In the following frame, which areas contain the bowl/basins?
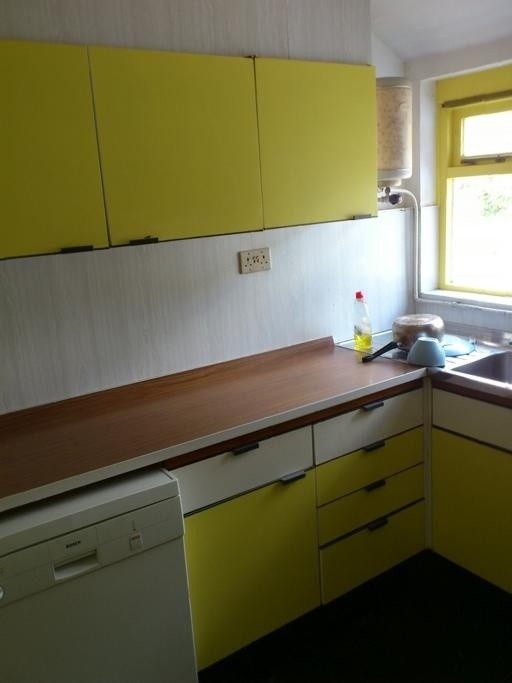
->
[407,337,446,367]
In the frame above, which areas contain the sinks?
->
[450,349,512,385]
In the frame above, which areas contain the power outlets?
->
[239,247,271,274]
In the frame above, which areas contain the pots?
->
[362,313,452,362]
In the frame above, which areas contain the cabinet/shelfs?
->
[312,379,426,604]
[0,43,265,259]
[423,377,510,595]
[253,55,379,230]
[171,421,322,674]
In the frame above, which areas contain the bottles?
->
[352,291,373,350]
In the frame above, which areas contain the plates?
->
[442,332,475,357]
